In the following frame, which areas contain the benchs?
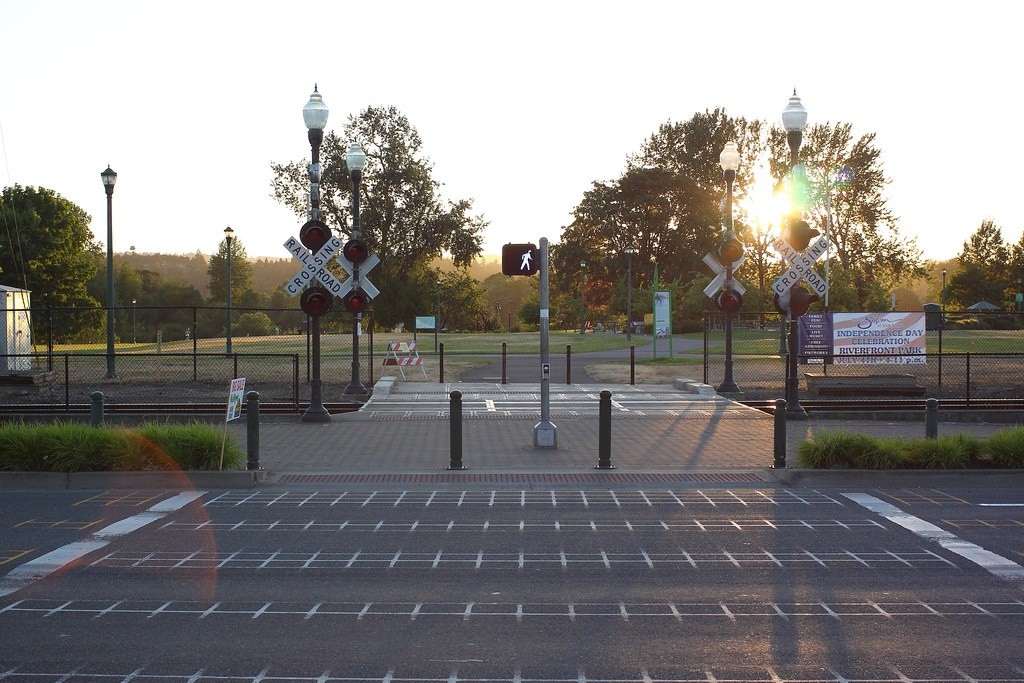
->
[803,372,927,399]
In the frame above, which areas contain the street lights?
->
[579,260,587,334]
[99,163,119,378]
[436,279,442,331]
[223,225,235,359]
[941,268,947,325]
[782,85,808,419]
[302,82,332,423]
[344,135,367,395]
[718,136,742,392]
[132,297,137,344]
[625,243,636,385]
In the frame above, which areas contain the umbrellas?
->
[966,299,1001,310]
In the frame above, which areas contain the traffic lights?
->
[715,289,742,312]
[500,242,539,277]
[718,239,743,263]
[790,221,820,251]
[791,286,820,317]
[343,239,368,262]
[301,287,333,318]
[299,220,334,251]
[343,290,367,314]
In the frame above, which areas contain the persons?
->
[184,328,190,340]
